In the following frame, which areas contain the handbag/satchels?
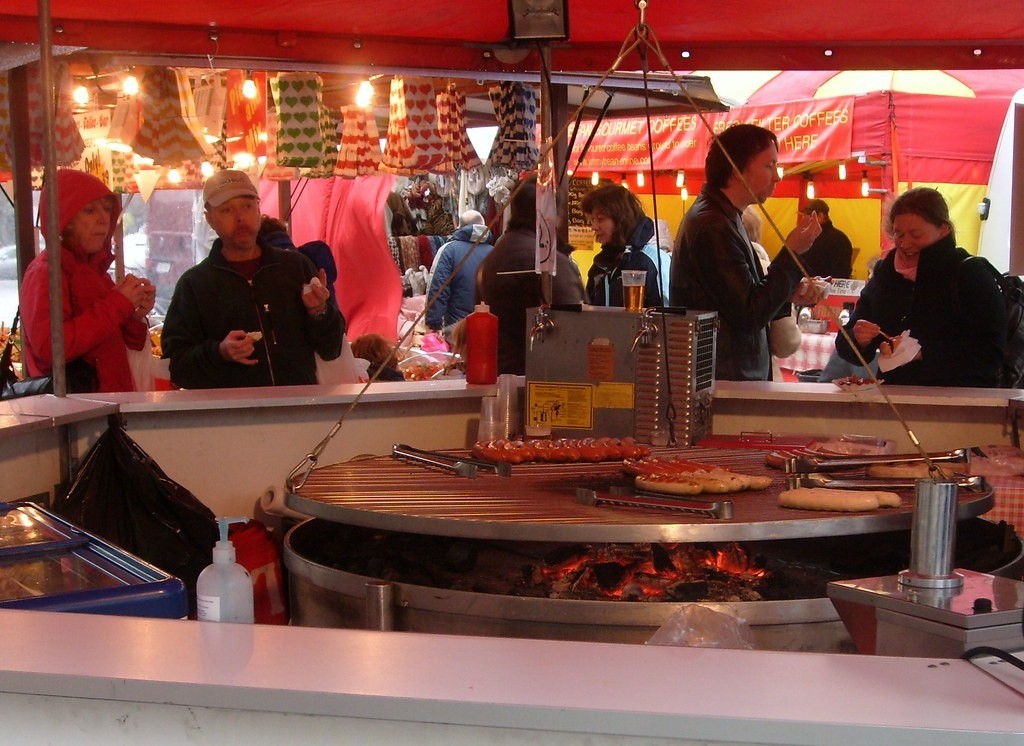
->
[0,342,97,401]
[954,256,1024,389]
[126,329,154,393]
[314,333,371,384]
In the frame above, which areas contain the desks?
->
[774,330,842,376]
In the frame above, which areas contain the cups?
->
[477,374,519,442]
[621,270,648,314]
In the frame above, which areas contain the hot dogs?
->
[801,275,832,286]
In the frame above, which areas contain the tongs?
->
[784,446,970,474]
[392,443,512,479]
[574,486,732,520]
[784,474,986,494]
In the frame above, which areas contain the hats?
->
[203,169,258,210]
[797,199,829,215]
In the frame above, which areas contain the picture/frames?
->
[508,0,571,42]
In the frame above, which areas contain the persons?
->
[582,185,671,306]
[472,177,587,375]
[669,125,834,381]
[350,334,405,382]
[741,205,771,275]
[835,187,993,387]
[797,198,853,278]
[160,170,346,388]
[425,210,494,333]
[19,170,156,393]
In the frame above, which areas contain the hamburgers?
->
[880,337,903,356]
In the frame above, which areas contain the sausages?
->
[473,437,953,509]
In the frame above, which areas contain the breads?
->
[245,331,263,343]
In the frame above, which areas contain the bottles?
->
[465,301,499,385]
